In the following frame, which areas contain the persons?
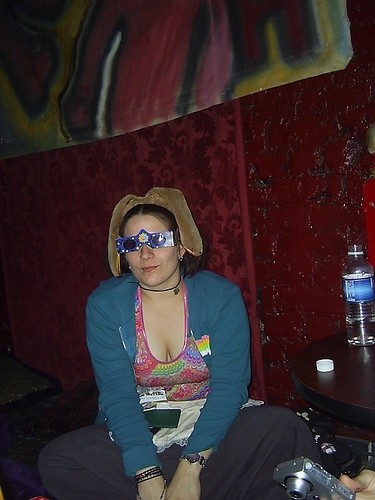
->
[332,458,375,500]
[38,187,321,500]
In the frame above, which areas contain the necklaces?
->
[137,273,182,295]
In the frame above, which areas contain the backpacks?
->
[291,405,363,477]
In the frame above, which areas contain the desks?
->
[290,332,375,445]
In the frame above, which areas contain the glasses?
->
[114,227,178,254]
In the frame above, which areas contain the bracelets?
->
[134,466,167,500]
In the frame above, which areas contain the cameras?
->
[273,456,356,500]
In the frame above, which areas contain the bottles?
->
[342,244,375,346]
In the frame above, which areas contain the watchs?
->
[179,451,207,468]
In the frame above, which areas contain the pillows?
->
[0,354,64,415]
